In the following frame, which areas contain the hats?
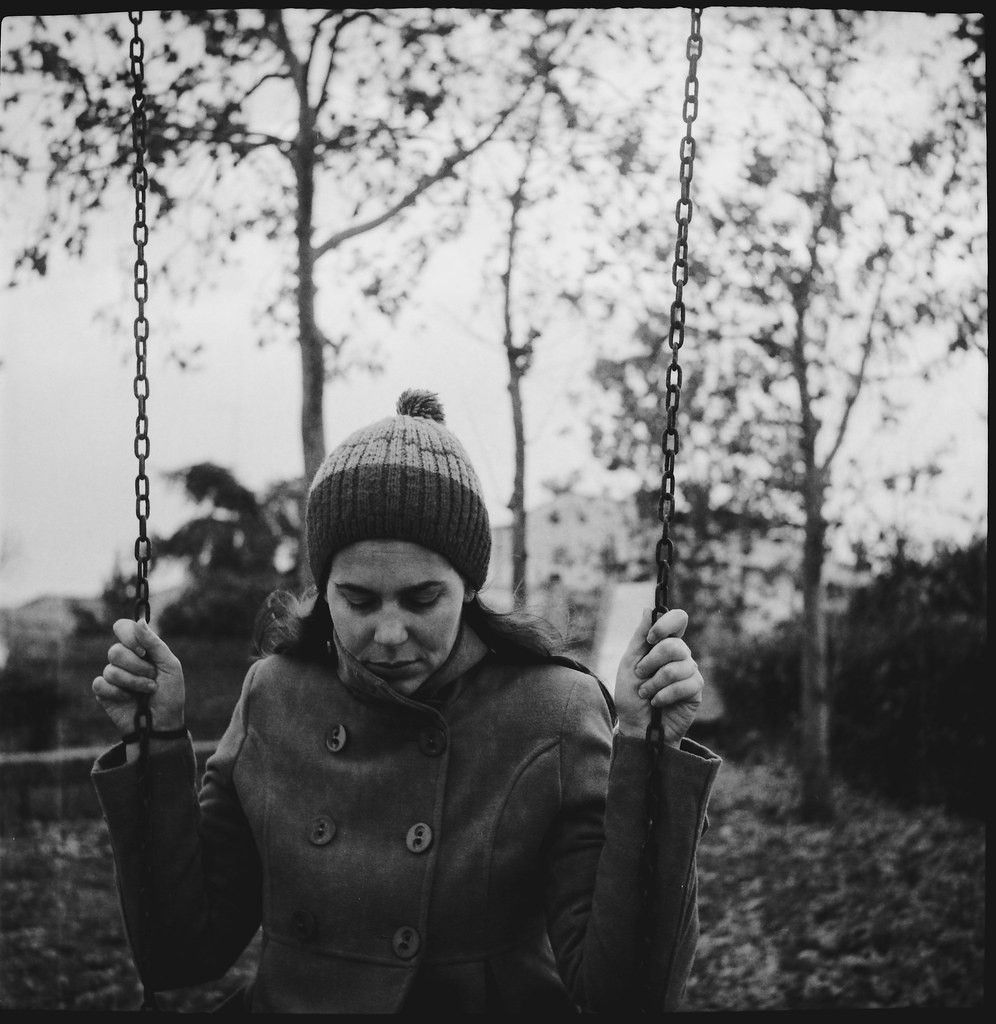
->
[306,389,491,593]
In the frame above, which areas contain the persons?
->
[90,387,722,1024]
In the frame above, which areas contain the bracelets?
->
[120,726,191,744]
